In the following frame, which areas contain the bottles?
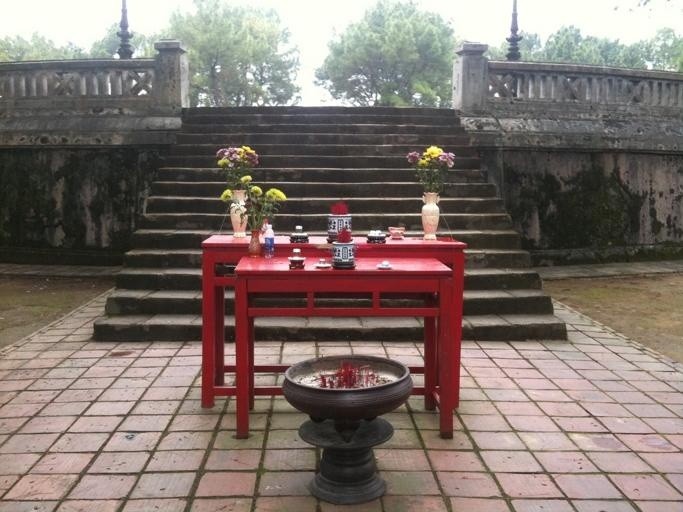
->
[264,225,275,259]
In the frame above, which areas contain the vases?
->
[326,215,354,241]
[247,229,263,258]
[421,192,440,240]
[332,241,357,268]
[229,190,249,238]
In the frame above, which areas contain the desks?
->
[199,230,467,409]
[235,253,458,440]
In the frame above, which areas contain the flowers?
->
[220,175,288,233]
[217,146,260,190]
[330,199,349,214]
[338,226,352,242]
[406,143,457,192]
